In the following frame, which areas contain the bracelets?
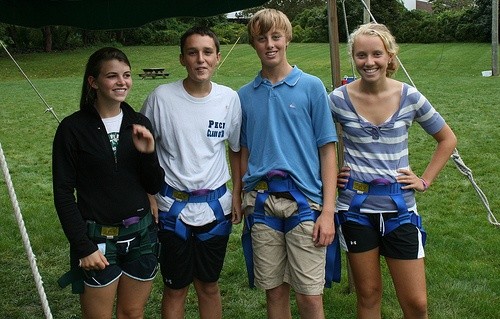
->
[419,176,429,189]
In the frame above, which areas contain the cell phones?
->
[97,243,106,256]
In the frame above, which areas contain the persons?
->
[54,47,166,319]
[328,24,457,319]
[238,9,338,318]
[140,27,241,319]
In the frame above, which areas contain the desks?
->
[142,68,164,73]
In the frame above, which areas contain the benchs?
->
[137,73,169,80]
